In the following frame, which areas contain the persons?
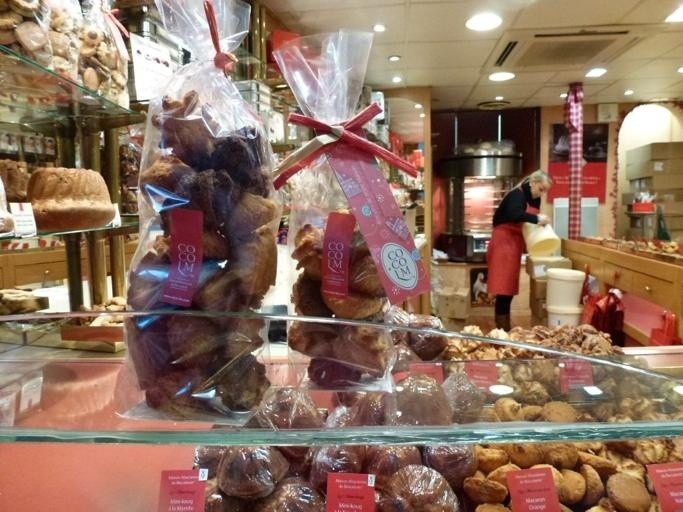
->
[487,169,553,332]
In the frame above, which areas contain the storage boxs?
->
[622,142,683,242]
[526,256,573,327]
[437,286,471,333]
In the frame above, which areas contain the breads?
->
[0,158,30,203]
[27,166,116,233]
[124,89,485,512]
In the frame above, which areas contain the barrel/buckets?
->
[546,267,586,306]
[545,306,583,328]
[522,221,561,257]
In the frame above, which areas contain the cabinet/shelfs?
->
[1,43,148,312]
[561,238,683,346]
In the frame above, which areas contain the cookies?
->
[445,324,683,512]
[0,0,127,92]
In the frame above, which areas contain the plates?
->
[552,148,606,160]
